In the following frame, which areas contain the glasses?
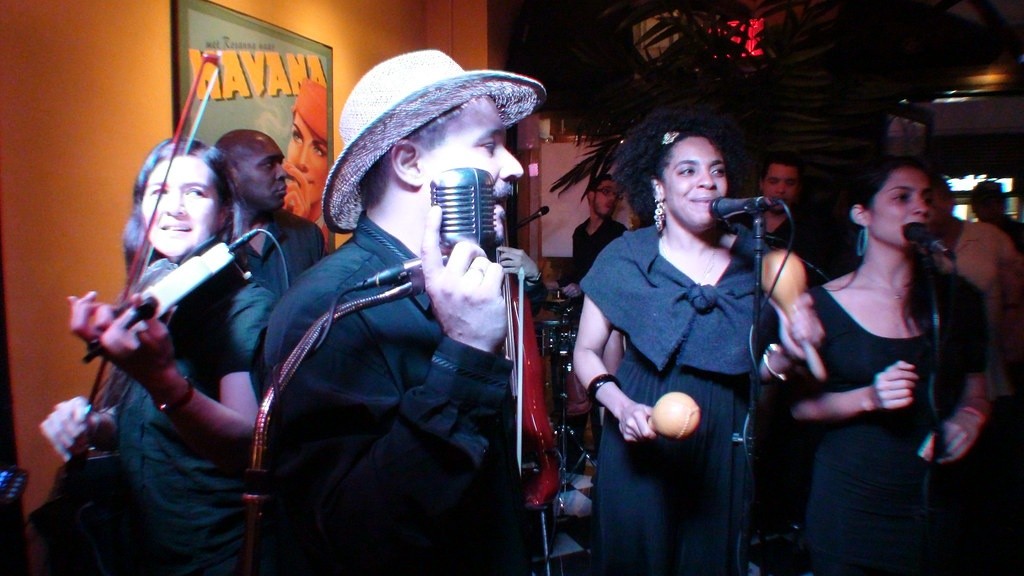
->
[592,187,624,201]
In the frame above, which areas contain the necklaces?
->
[661,233,716,283]
[856,269,910,300]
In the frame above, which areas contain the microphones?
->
[708,195,781,220]
[515,205,550,231]
[355,168,496,292]
[903,221,958,262]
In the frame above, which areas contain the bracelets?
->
[956,404,987,420]
[585,374,621,408]
[150,375,193,414]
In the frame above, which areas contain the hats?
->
[321,49,545,234]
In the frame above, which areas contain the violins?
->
[80,241,235,365]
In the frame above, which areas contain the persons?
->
[39,135,278,576]
[213,130,327,301]
[498,115,1024,576]
[249,50,547,576]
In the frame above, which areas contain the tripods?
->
[543,357,597,525]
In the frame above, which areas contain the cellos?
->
[500,263,564,576]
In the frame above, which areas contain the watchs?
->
[528,271,543,284]
